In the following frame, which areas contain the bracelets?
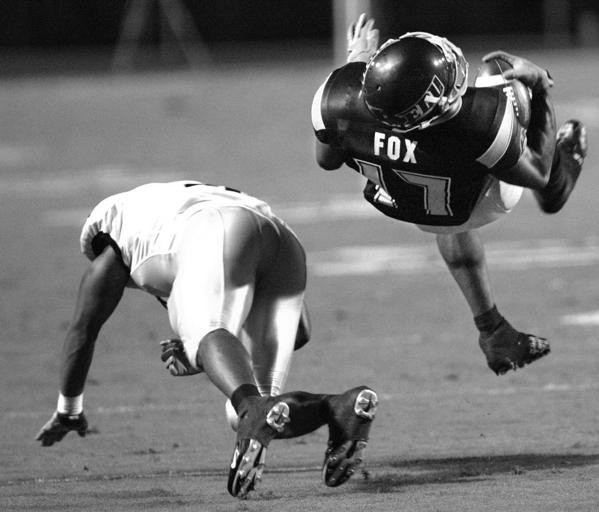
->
[56,388,84,416]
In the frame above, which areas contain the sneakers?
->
[320,384,381,487]
[478,317,551,377]
[225,393,291,500]
[530,119,589,215]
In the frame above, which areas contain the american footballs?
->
[474,58,532,129]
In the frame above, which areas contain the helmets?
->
[360,36,456,132]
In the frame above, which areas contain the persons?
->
[308,10,590,377]
[34,179,379,500]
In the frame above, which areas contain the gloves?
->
[34,393,90,449]
[481,48,553,93]
[159,340,204,378]
[345,13,381,66]
